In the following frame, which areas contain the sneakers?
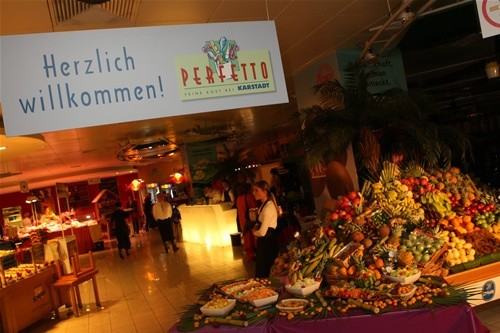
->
[165,244,179,253]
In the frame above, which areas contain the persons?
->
[109,195,138,259]
[144,168,302,278]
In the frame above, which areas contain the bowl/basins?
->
[285,281,320,298]
[247,291,279,309]
[385,269,421,283]
[200,298,236,317]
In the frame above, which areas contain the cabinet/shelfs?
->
[0,263,58,333]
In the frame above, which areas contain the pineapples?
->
[429,162,489,207]
[373,243,415,270]
[352,255,376,287]
[388,223,406,248]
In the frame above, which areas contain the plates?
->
[275,298,309,313]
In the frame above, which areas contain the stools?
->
[50,250,101,318]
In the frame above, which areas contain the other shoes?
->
[120,252,129,259]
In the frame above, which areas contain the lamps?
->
[486,58,500,79]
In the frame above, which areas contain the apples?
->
[326,191,360,222]
[399,176,440,200]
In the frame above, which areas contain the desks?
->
[167,277,491,332]
[48,224,98,254]
